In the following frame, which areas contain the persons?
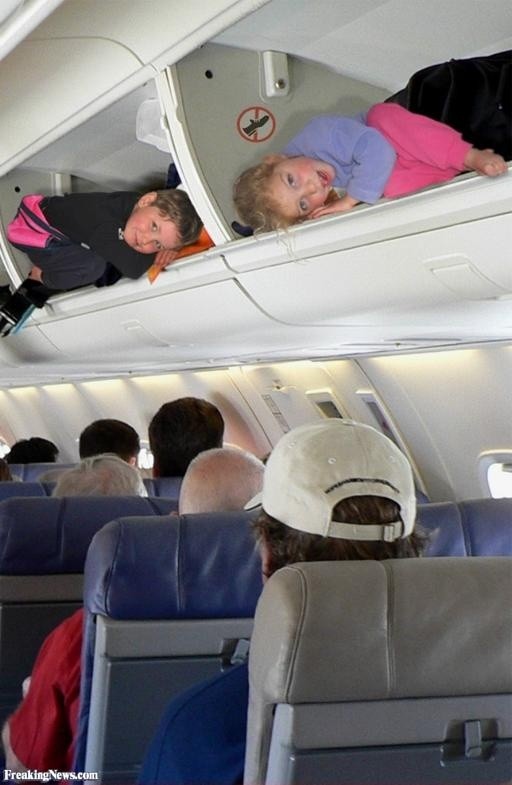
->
[9,438,58,469]
[1,189,200,335]
[148,397,225,477]
[3,445,266,784]
[80,418,141,472]
[234,48,512,227]
[51,455,146,501]
[144,418,421,785]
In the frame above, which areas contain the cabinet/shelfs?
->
[167,2,512,246]
[0,69,219,300]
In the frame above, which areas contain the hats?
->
[242,418,418,542]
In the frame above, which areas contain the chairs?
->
[0,495,188,721]
[74,495,511,785]
[241,558,512,785]
